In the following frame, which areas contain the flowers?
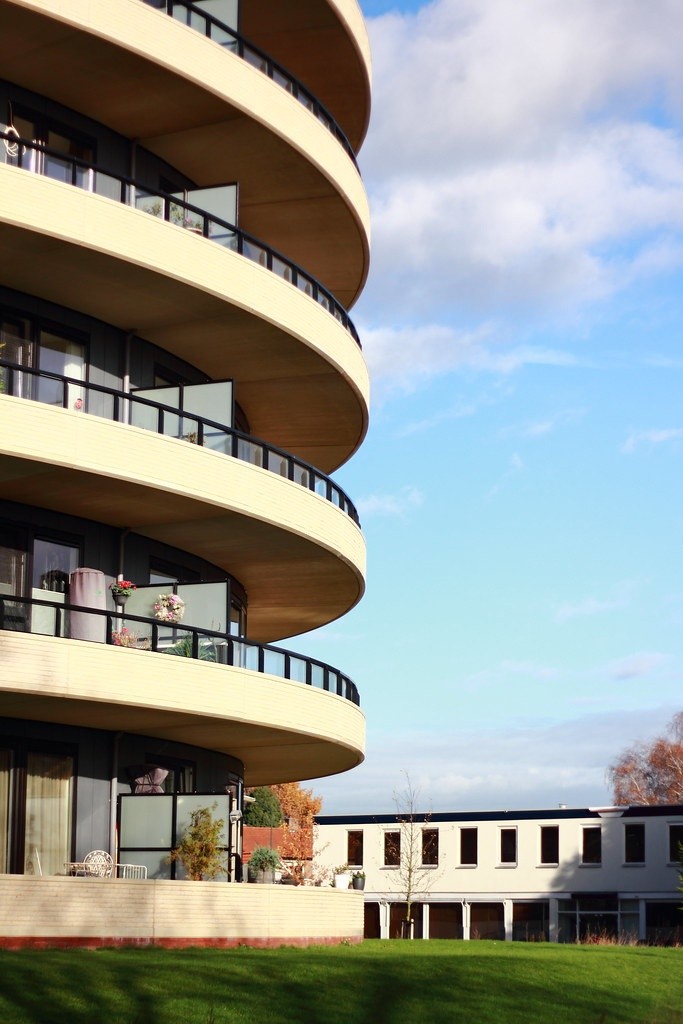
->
[152,593,185,624]
[110,580,136,597]
[110,625,152,650]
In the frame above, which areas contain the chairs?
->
[83,850,112,878]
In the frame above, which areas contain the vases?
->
[112,594,129,605]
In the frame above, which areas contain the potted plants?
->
[334,866,350,889]
[185,220,202,236]
[353,870,365,891]
[281,871,300,886]
